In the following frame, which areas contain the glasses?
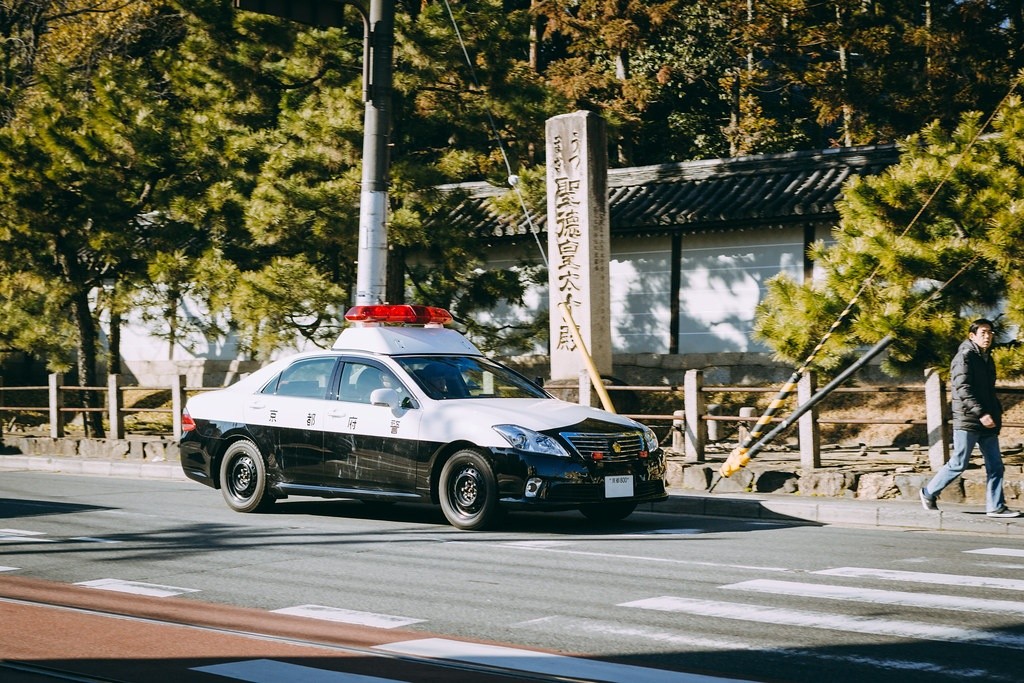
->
[973,331,995,336]
[431,376,447,382]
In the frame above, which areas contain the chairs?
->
[354,367,385,404]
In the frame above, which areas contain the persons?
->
[919,318,1021,518]
[366,364,458,409]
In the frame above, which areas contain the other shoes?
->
[919,488,939,510]
[987,508,1021,518]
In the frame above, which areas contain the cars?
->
[179,303,669,532]
